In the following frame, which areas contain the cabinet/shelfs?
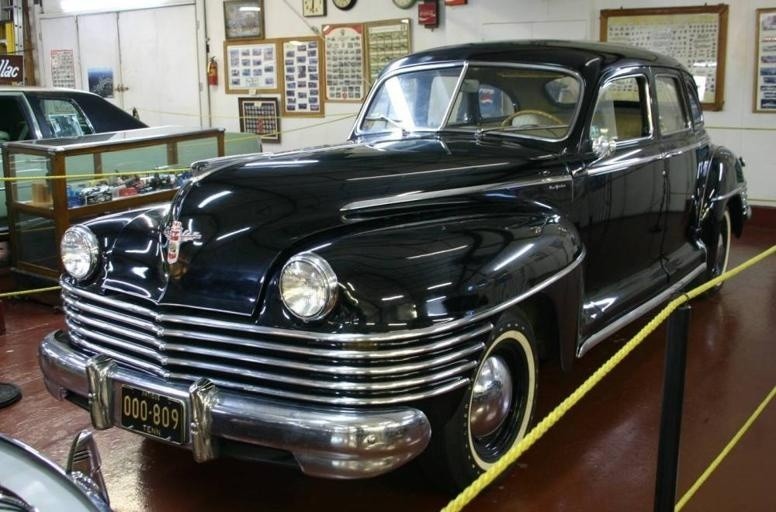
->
[3,124,225,312]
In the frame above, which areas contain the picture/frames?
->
[599,3,729,112]
[750,8,776,114]
[223,0,413,144]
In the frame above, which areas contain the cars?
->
[35,32,758,486]
[0,86,266,243]
[0,429,116,512]
